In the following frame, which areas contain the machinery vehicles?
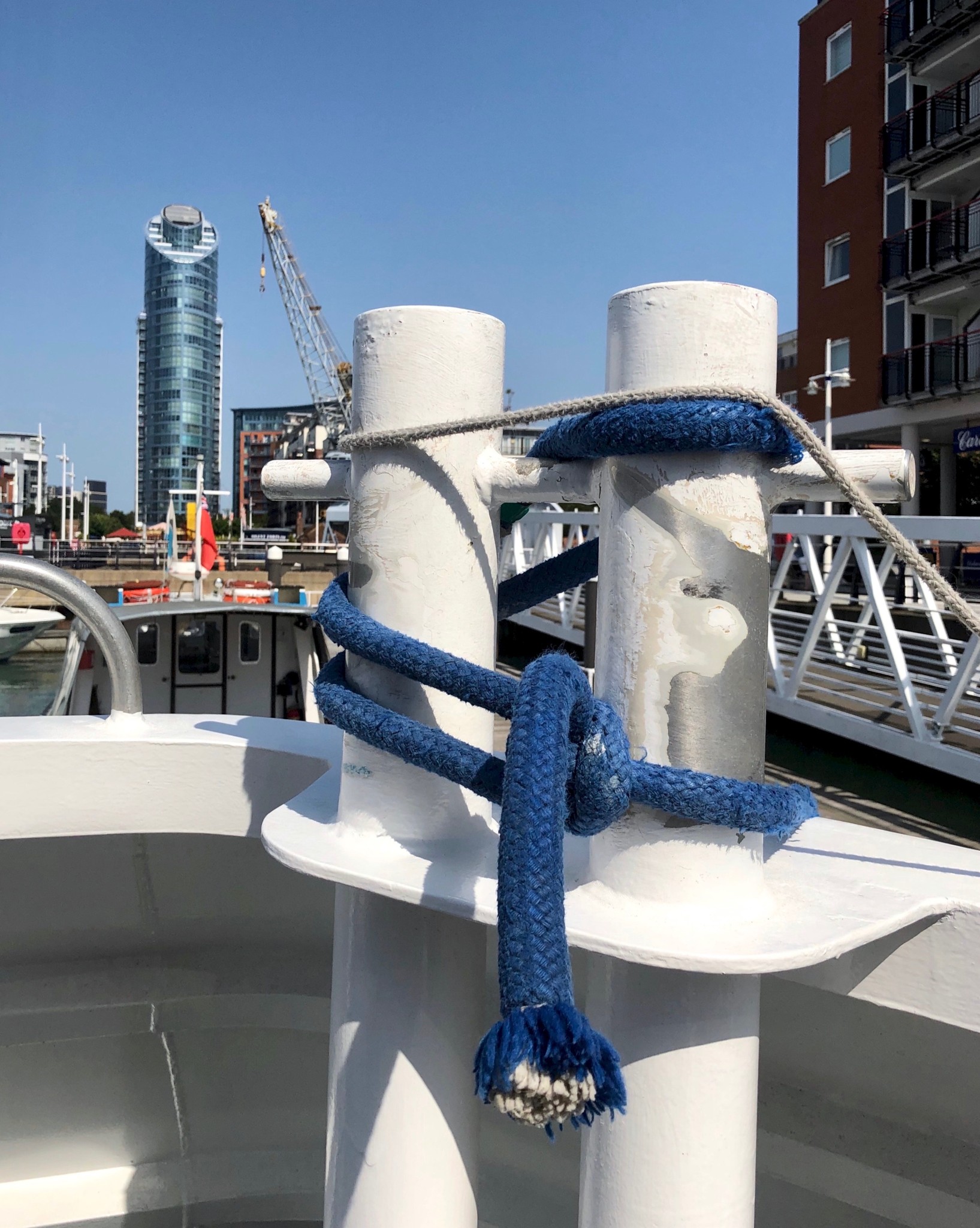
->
[258,195,352,447]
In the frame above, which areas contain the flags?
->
[196,482,218,572]
[164,498,178,574]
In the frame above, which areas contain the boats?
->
[168,559,210,583]
[0,606,66,662]
